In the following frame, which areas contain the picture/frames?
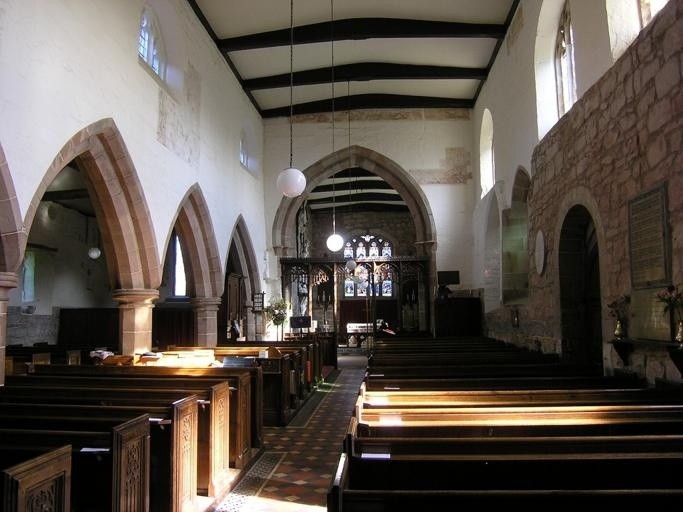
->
[627,178,670,289]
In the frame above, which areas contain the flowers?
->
[601,287,683,319]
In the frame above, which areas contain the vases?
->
[612,320,683,343]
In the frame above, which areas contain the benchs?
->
[325,327,682,512]
[1,329,341,512]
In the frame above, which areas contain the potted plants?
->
[267,301,287,325]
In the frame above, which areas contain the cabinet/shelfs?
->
[57,273,246,351]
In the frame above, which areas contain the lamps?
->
[88,212,102,259]
[275,0,357,276]
